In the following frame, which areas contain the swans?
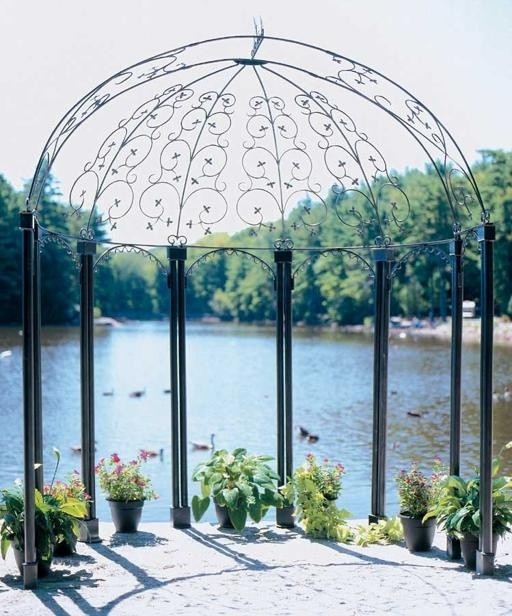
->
[140,447,164,458]
[190,431,217,450]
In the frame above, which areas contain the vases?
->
[54,538,73,556]
[397,511,435,550]
[106,497,144,534]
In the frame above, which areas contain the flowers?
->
[394,453,448,520]
[94,449,160,502]
[42,447,94,509]
[304,451,346,500]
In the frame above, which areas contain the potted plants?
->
[0,482,92,579]
[193,448,296,530]
[421,438,512,572]
